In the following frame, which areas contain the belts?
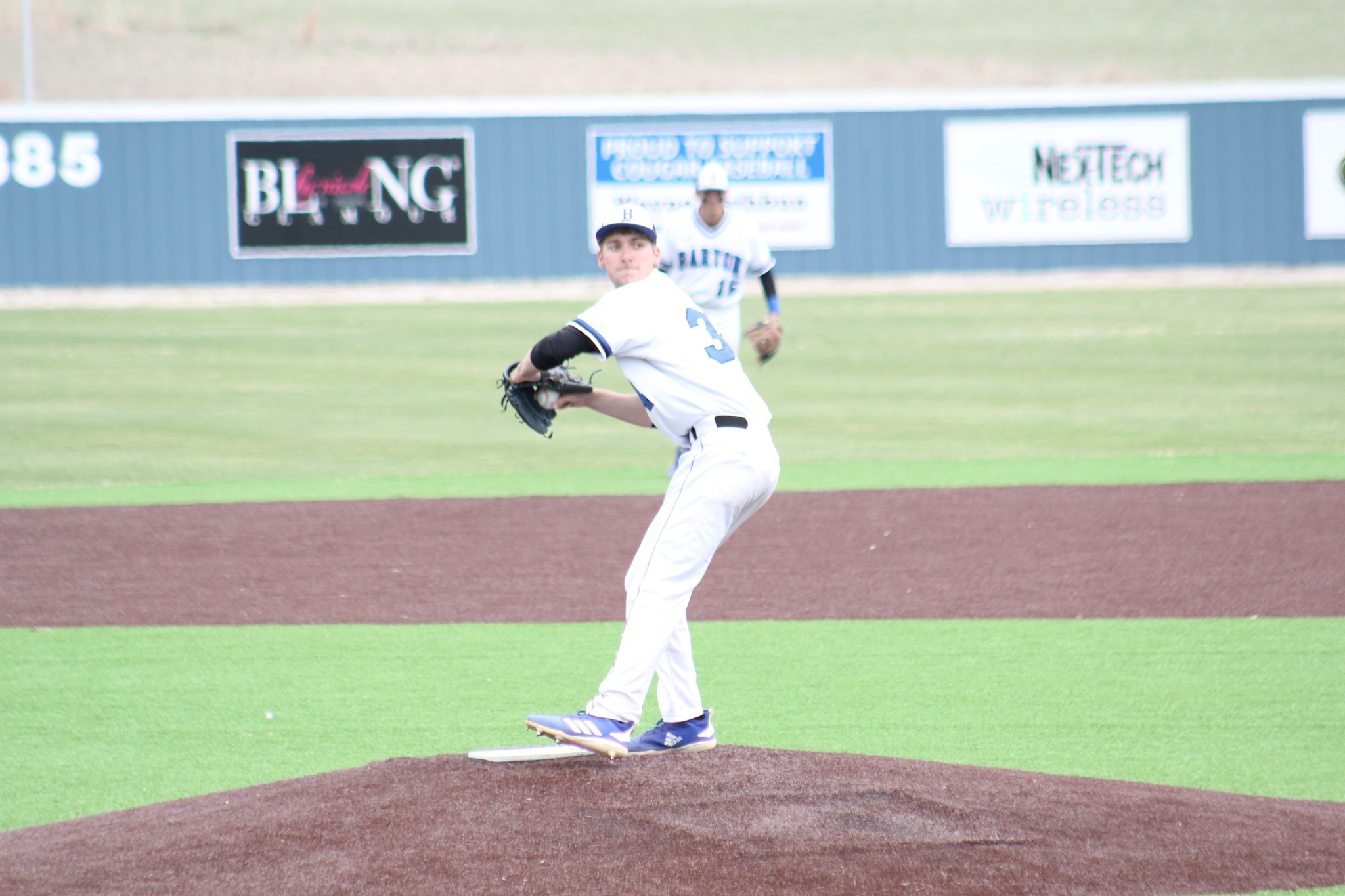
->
[686,415,747,443]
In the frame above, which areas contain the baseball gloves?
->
[744,320,783,364]
[502,361,594,435]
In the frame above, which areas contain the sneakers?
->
[628,709,716,755]
[526,710,638,759]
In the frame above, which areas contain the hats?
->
[596,203,657,247]
[695,161,729,192]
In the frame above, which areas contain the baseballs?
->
[537,386,561,410]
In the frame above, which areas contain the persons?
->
[655,160,780,376]
[500,203,779,762]
